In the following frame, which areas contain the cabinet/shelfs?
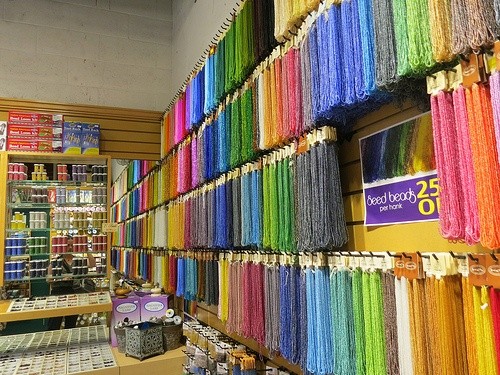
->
[1,149,112,288]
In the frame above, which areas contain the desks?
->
[0,291,113,327]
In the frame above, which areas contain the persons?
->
[48,251,97,332]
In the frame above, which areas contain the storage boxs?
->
[109,287,184,362]
[0,111,100,154]
